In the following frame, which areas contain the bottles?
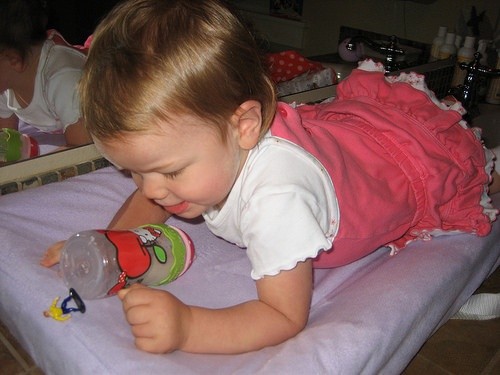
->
[431,26,448,59]
[59,223,195,299]
[454,35,462,49]
[452,37,477,88]
[441,33,456,60]
[0,128,40,164]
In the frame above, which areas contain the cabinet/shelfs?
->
[237,8,341,57]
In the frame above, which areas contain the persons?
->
[0,0,95,154]
[43,288,85,322]
[42,0,500,355]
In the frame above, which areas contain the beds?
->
[18,25,433,156]
[0,58,500,374]
